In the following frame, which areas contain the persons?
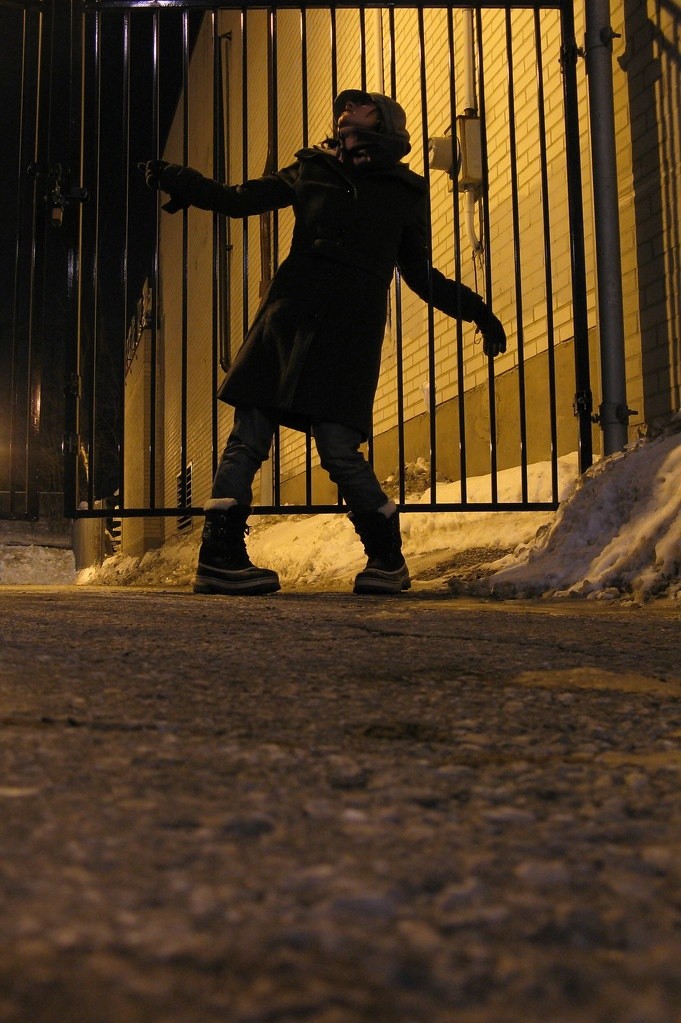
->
[138,90,506,596]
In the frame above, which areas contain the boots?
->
[347,502,412,594]
[194,499,281,594]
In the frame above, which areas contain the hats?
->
[333,89,410,162]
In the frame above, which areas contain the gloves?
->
[474,302,506,356]
[138,158,187,194]
